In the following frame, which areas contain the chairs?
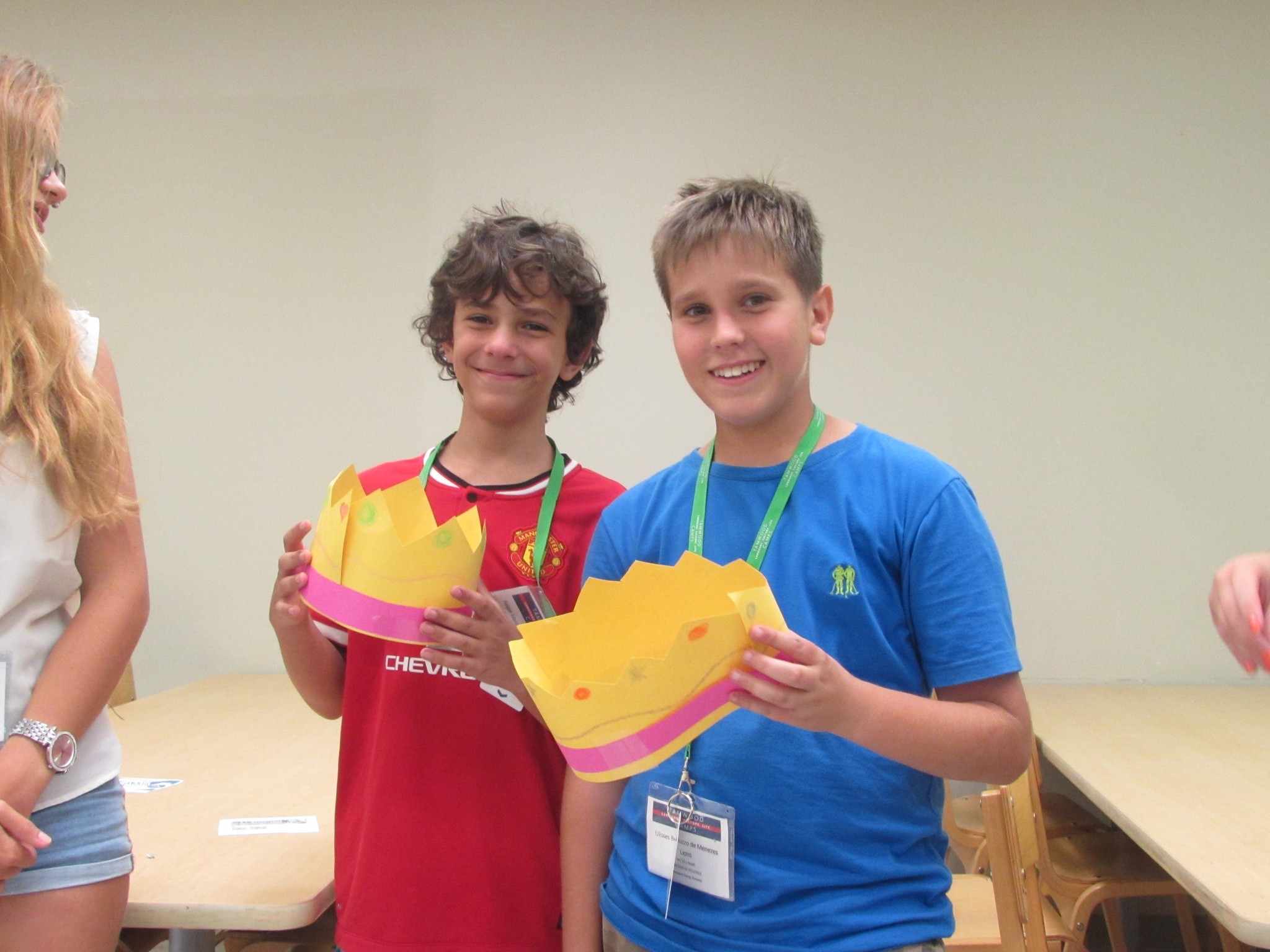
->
[943,730,1202,952]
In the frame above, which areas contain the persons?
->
[561,177,1031,952]
[269,216,629,952]
[0,54,150,952]
[1209,553,1270,675]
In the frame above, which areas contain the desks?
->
[107,671,340,952]
[1020,683,1270,951]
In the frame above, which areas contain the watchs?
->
[8,718,76,775]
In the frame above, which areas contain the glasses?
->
[28,128,65,208]
